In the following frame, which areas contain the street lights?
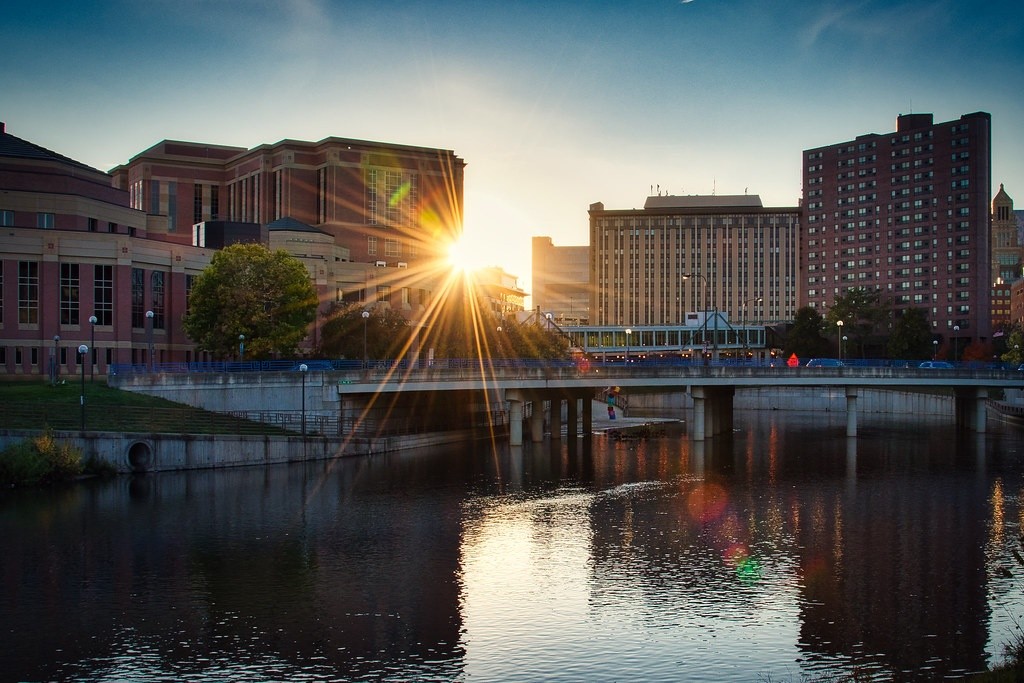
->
[78,344,89,431]
[545,312,552,360]
[239,334,244,373]
[842,335,848,360]
[836,320,843,361]
[681,274,708,367]
[299,364,308,435]
[626,329,633,359]
[362,311,369,371]
[145,311,154,366]
[88,316,98,385]
[742,297,763,367]
[954,325,960,362]
[933,340,937,359]
[496,326,503,357]
[54,335,61,384]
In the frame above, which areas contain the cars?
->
[919,361,955,368]
[806,358,846,367]
